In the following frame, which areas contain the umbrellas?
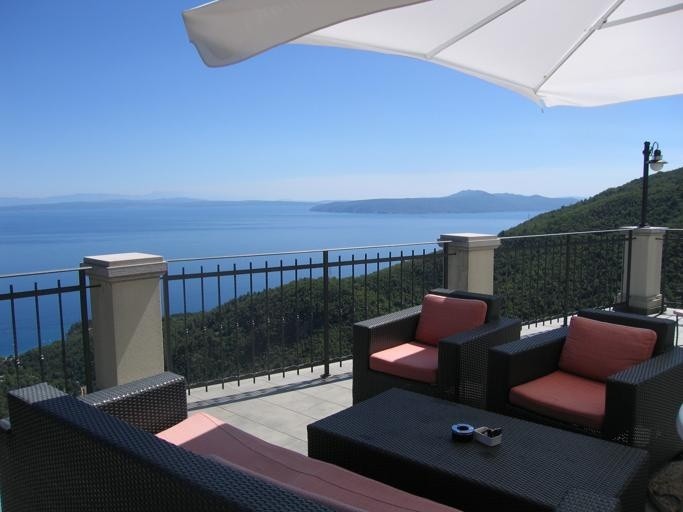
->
[181,0,683,109]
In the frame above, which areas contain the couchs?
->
[490,308,682,474]
[1,372,619,511]
[350,287,520,411]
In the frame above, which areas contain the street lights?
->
[638,141,670,229]
